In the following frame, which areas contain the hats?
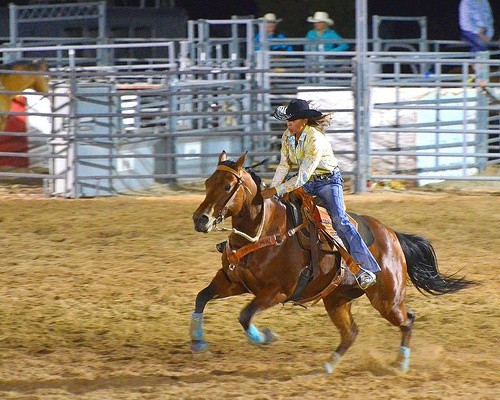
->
[274,99,322,121]
[307,11,334,25]
[258,12,283,23]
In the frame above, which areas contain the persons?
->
[252,13,292,78]
[261,99,381,288]
[459,0,494,60]
[304,11,348,83]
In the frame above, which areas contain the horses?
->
[190,150,484,374]
[0,56,49,130]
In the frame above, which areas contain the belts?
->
[308,168,340,182]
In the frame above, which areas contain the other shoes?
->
[360,272,377,287]
